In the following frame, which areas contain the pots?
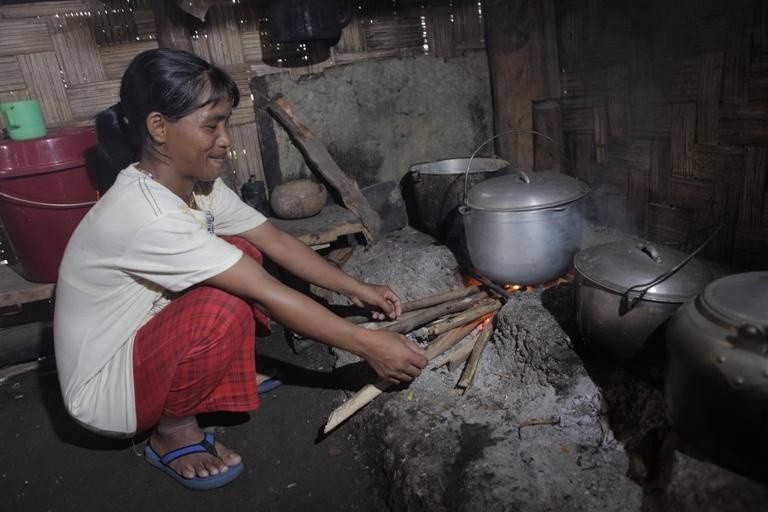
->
[458,127,592,286]
[573,239,714,373]
[662,270,768,486]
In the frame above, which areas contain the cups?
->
[0,98,47,142]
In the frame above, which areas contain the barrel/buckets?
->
[1,125,99,284]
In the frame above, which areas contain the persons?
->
[53,46,431,490]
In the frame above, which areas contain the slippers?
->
[257,367,281,393]
[145,433,245,490]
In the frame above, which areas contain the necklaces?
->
[136,166,197,210]
